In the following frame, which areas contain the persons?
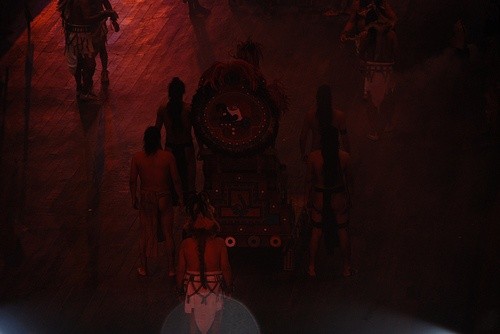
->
[58,0,119,103]
[176,193,237,334]
[130,127,182,277]
[185,0,210,19]
[298,86,351,158]
[350,1,398,142]
[300,128,353,278]
[155,77,206,217]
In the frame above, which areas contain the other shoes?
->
[101,70,110,89]
[168,265,176,277]
[137,267,148,276]
[75,87,82,96]
[79,92,97,100]
